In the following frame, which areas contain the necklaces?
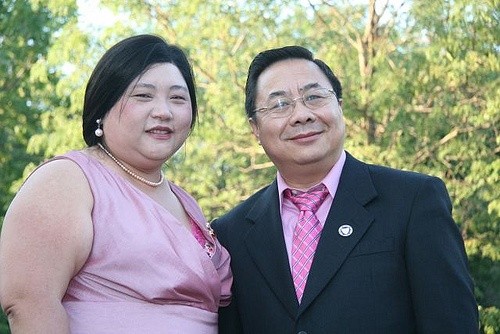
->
[97,141,165,186]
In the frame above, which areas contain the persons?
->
[1,33,234,333]
[207,45,484,334]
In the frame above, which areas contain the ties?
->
[283,182,329,306]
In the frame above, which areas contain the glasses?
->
[252,88,339,117]
